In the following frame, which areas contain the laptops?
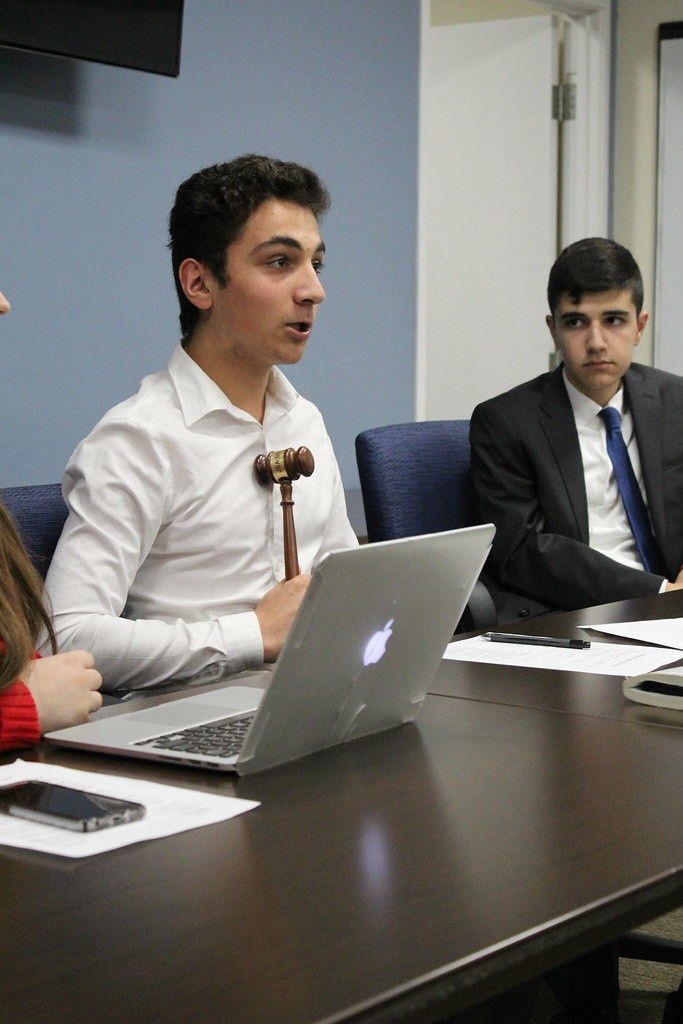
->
[41,522,496,777]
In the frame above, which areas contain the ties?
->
[598,408,664,577]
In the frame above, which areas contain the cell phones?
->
[0,780,146,833]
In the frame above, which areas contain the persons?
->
[0,291,104,747]
[36,153,359,701]
[467,237,683,626]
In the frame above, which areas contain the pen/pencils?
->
[481,632,590,649]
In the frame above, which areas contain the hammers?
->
[253,446,316,581]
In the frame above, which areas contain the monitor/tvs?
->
[0,0,184,77]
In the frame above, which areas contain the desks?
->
[0,588,683,1024]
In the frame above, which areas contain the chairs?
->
[0,484,69,582]
[354,420,501,634]
